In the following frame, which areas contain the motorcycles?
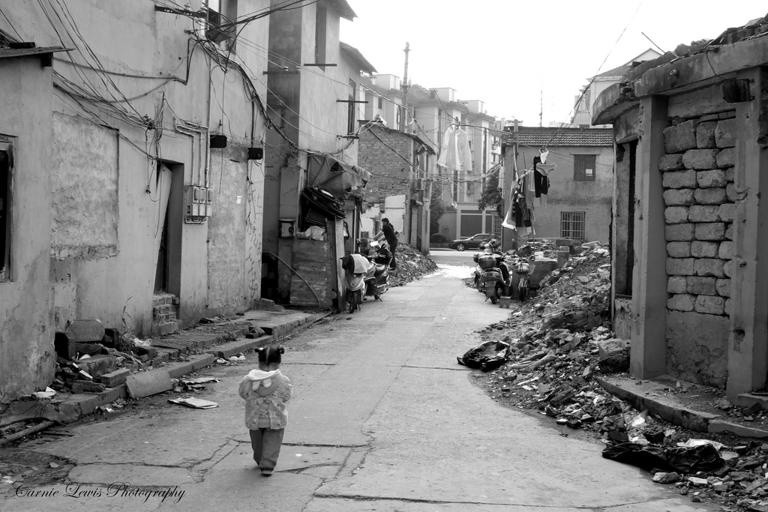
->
[337,236,394,312]
[472,238,535,306]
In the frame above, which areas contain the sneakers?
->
[260,462,275,475]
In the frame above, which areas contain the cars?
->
[449,230,499,252]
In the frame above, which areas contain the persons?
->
[239,346,293,479]
[374,218,399,270]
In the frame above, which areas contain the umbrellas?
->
[342,253,370,273]
